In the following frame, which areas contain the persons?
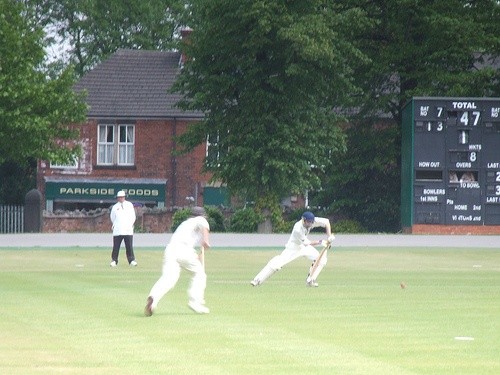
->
[110,191,136,266]
[250,212,332,286]
[144,208,211,316]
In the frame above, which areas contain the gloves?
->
[319,239,327,247]
[328,235,335,242]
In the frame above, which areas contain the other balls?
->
[401,282,407,288]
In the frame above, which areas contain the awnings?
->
[203,187,231,205]
[43,176,167,201]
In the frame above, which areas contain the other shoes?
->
[251,280,257,286]
[130,261,137,266]
[110,261,116,266]
[145,296,154,316]
[306,282,318,287]
[188,301,210,314]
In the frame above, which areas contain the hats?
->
[117,190,125,197]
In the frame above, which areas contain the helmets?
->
[301,212,315,223]
[189,206,204,216]
